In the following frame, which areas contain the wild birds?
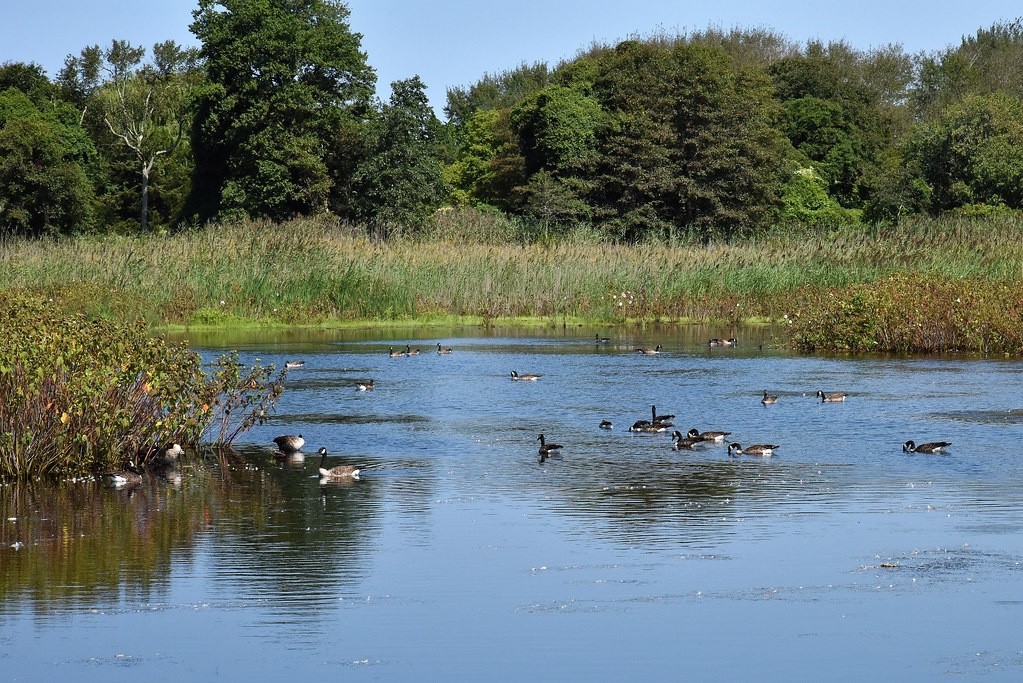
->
[149,443,187,467]
[598,419,614,429]
[816,390,848,402]
[635,344,663,355]
[510,371,543,381]
[285,360,305,368]
[628,404,675,433]
[671,428,732,449]
[903,440,953,455]
[728,442,781,457]
[594,333,610,343]
[708,337,738,347]
[436,342,455,354]
[388,344,422,358]
[102,458,143,484]
[315,447,366,477]
[536,433,565,454]
[761,389,778,404]
[354,379,377,392]
[272,433,306,453]
